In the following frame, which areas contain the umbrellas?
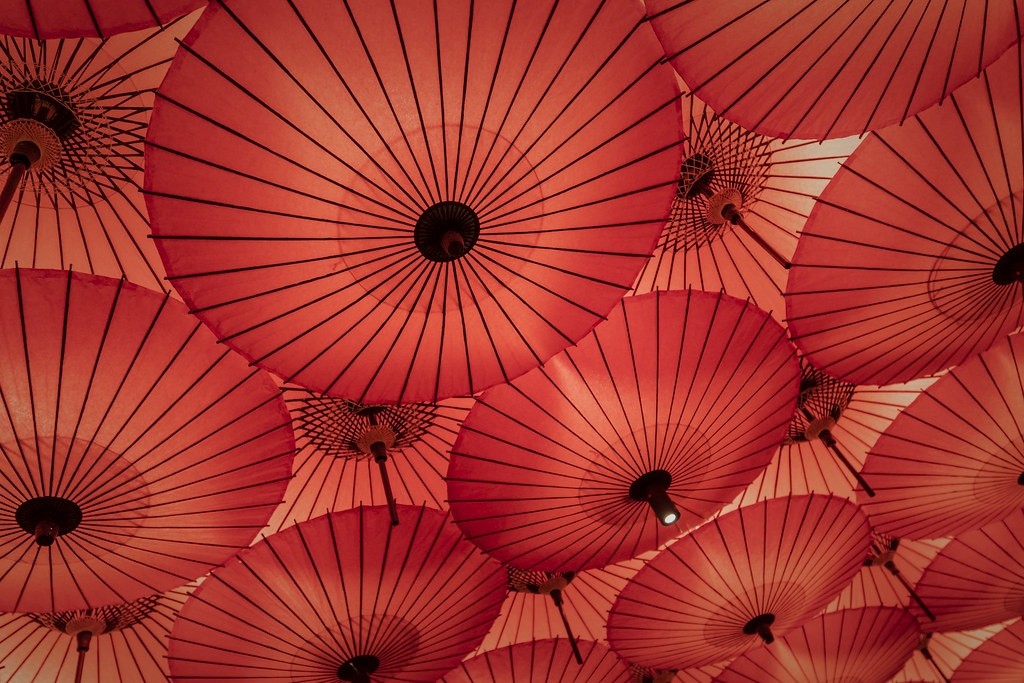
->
[1,370,1024,683]
[144,1,684,404]
[443,285,802,573]
[0,1,210,305]
[623,0,1023,385]
[0,268,295,612]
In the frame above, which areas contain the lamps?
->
[630,467,681,526]
